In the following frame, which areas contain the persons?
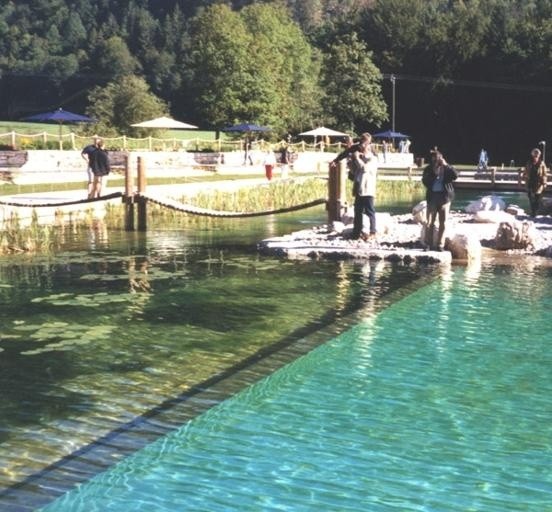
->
[81,137,104,199]
[422,149,458,253]
[345,137,354,161]
[330,132,378,214]
[341,142,378,240]
[396,138,411,153]
[381,140,389,163]
[519,147,550,220]
[242,135,253,165]
[85,140,110,199]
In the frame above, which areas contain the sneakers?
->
[424,244,431,251]
[438,246,444,252]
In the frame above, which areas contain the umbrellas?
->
[17,107,100,140]
[297,125,350,141]
[129,115,199,128]
[222,121,272,136]
[373,128,411,143]
